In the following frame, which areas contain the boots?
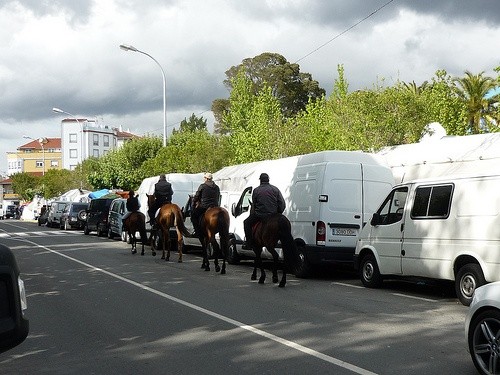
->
[121,218,128,231]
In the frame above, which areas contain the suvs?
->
[60,203,90,230]
[84,199,113,237]
[47,202,66,228]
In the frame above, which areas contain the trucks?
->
[0,203,19,220]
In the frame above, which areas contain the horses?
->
[122,212,147,255]
[146,203,192,263]
[188,194,231,275]
[243,198,301,289]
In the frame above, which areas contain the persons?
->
[241,172,286,250]
[11,207,22,219]
[190,172,220,239]
[122,190,141,232]
[148,173,174,226]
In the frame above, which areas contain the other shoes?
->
[193,232,200,237]
[241,244,253,250]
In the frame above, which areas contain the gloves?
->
[191,202,197,208]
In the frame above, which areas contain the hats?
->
[160,174,166,179]
[259,173,269,182]
[127,190,135,195]
[204,173,212,179]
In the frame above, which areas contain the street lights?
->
[23,135,45,191]
[119,43,167,147]
[51,107,83,190]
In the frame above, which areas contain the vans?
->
[353,174,500,306]
[126,174,218,250]
[178,191,251,259]
[107,198,126,242]
[229,151,399,278]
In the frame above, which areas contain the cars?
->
[464,281,500,375]
[0,244,31,354]
[38,210,50,226]
